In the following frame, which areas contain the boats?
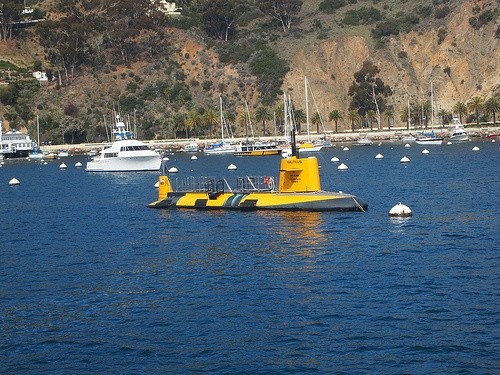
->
[183,143,200,153]
[1,115,42,158]
[84,101,164,171]
[352,137,374,147]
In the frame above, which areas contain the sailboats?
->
[147,94,368,215]
[203,75,336,155]
[390,79,500,146]
